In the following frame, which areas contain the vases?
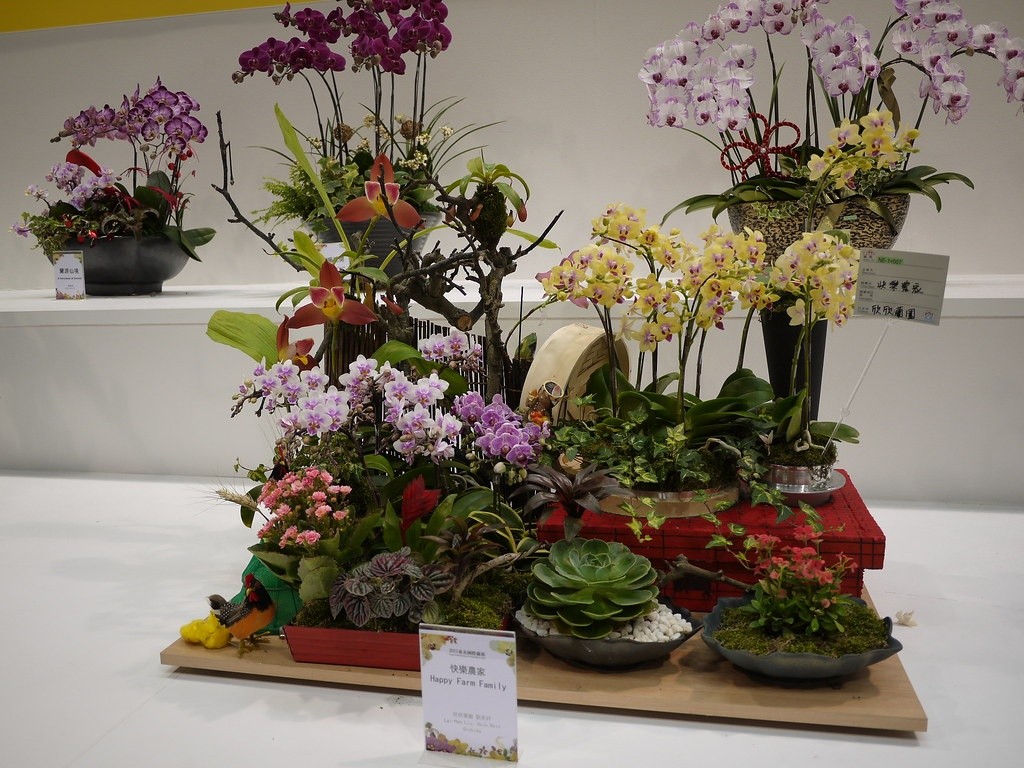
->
[699,592,903,681]
[628,442,730,492]
[305,214,442,292]
[758,463,846,507]
[724,194,911,265]
[45,236,189,297]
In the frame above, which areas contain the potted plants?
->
[511,537,703,672]
[282,461,620,672]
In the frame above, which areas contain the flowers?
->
[206,1,1024,612]
[5,75,217,263]
